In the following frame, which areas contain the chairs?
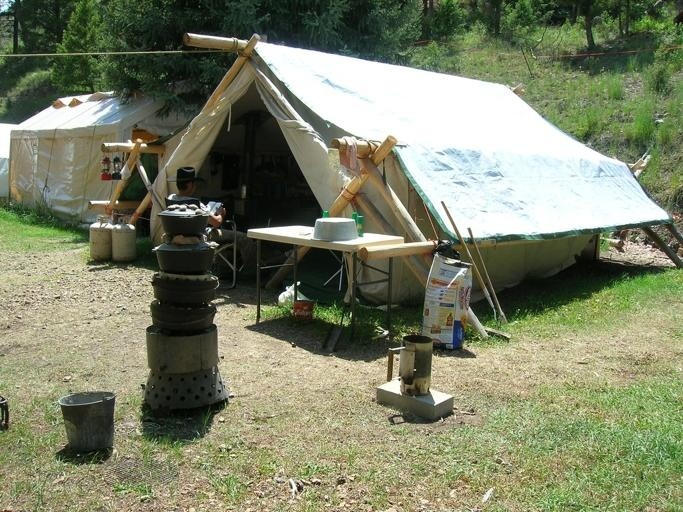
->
[164,198,238,292]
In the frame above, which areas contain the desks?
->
[248,224,405,344]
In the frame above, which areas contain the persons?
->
[165,166,263,278]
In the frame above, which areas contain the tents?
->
[148,39,673,313]
[9,78,217,229]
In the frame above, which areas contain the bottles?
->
[351,212,365,237]
[322,210,329,218]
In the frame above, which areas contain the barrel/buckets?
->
[59,390,116,453]
[294,299,315,322]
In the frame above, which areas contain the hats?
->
[167,167,208,182]
